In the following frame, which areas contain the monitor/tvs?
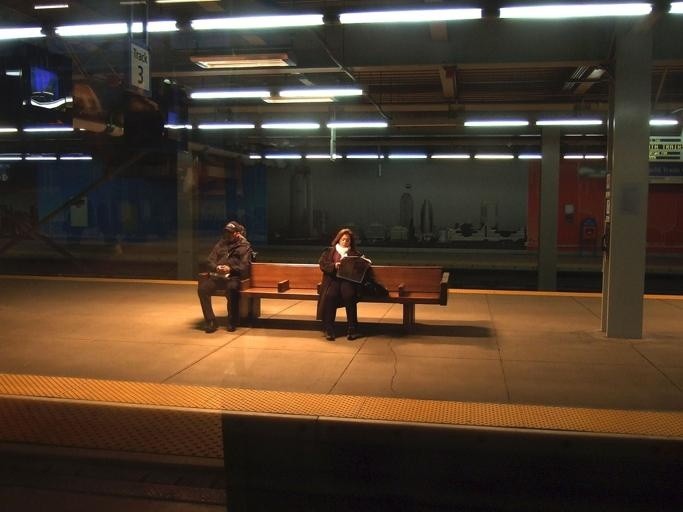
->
[159,83,188,162]
[17,43,72,126]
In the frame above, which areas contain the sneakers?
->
[226,320,235,332]
[324,325,336,341]
[346,325,357,341]
[204,319,219,333]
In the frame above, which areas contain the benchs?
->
[210,262,450,331]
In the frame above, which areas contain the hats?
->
[223,220,241,233]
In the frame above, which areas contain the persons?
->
[197,222,251,332]
[315,228,388,341]
